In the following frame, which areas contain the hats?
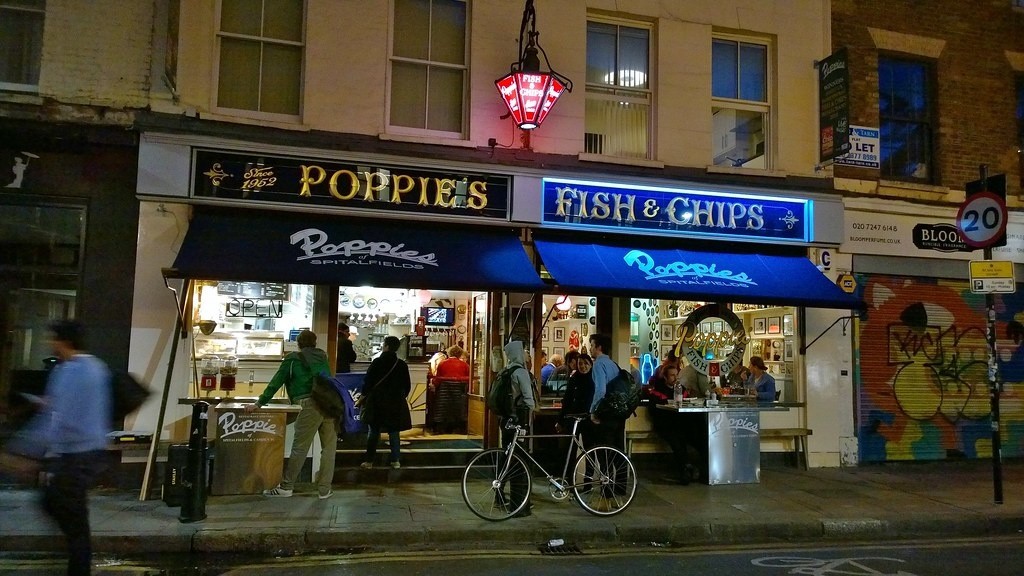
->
[349,326,359,336]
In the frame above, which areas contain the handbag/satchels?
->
[592,360,641,429]
[354,395,366,407]
[298,351,346,418]
[112,370,150,420]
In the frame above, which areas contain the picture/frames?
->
[541,326,566,359]
[660,313,795,364]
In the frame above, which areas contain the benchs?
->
[625,427,814,477]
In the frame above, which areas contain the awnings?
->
[533,232,868,349]
[161,205,544,340]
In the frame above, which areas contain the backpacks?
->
[485,365,533,416]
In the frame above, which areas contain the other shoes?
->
[590,499,612,511]
[584,488,593,494]
[493,499,510,507]
[612,495,624,508]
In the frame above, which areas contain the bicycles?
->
[461,412,637,522]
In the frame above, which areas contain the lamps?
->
[192,318,217,336]
[495,0,573,129]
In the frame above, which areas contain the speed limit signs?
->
[955,194,1008,249]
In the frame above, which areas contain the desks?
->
[106,437,176,451]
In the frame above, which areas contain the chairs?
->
[427,381,468,432]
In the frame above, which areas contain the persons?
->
[244,331,338,498]
[337,323,357,374]
[494,341,539,513]
[17,320,107,576]
[359,335,412,469]
[647,346,776,475]
[431,346,471,385]
[541,350,581,394]
[560,335,627,510]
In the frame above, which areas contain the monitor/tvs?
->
[425,307,455,326]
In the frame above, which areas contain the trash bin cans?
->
[165,438,215,507]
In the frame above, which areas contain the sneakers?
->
[391,462,401,469]
[319,489,336,500]
[263,483,293,498]
[360,462,372,469]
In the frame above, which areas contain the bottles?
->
[722,380,756,395]
[249,371,254,382]
[674,381,683,408]
[642,353,654,385]
